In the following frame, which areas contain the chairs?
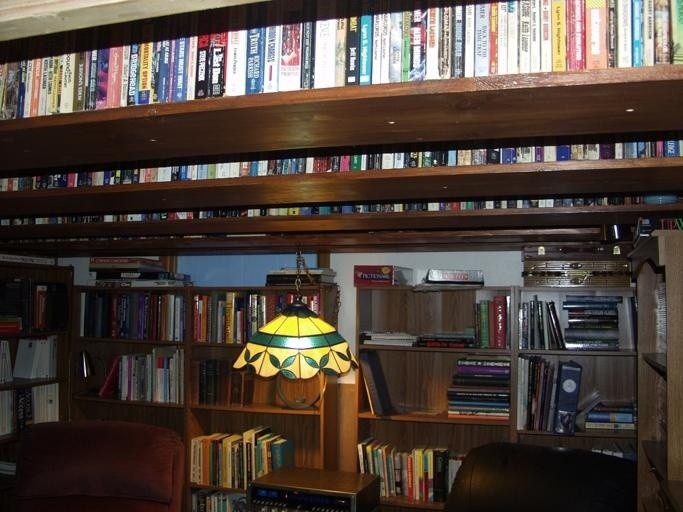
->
[2,418,188,510]
[444,438,639,511]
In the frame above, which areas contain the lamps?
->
[233,240,362,381]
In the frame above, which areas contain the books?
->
[0,0,683,119]
[357,270,637,504]
[1,141,683,246]
[1,255,321,512]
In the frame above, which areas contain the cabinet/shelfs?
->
[189,284,338,511]
[352,283,514,509]
[638,230,683,512]
[518,284,642,461]
[2,261,72,436]
[247,468,380,511]
[76,283,184,434]
